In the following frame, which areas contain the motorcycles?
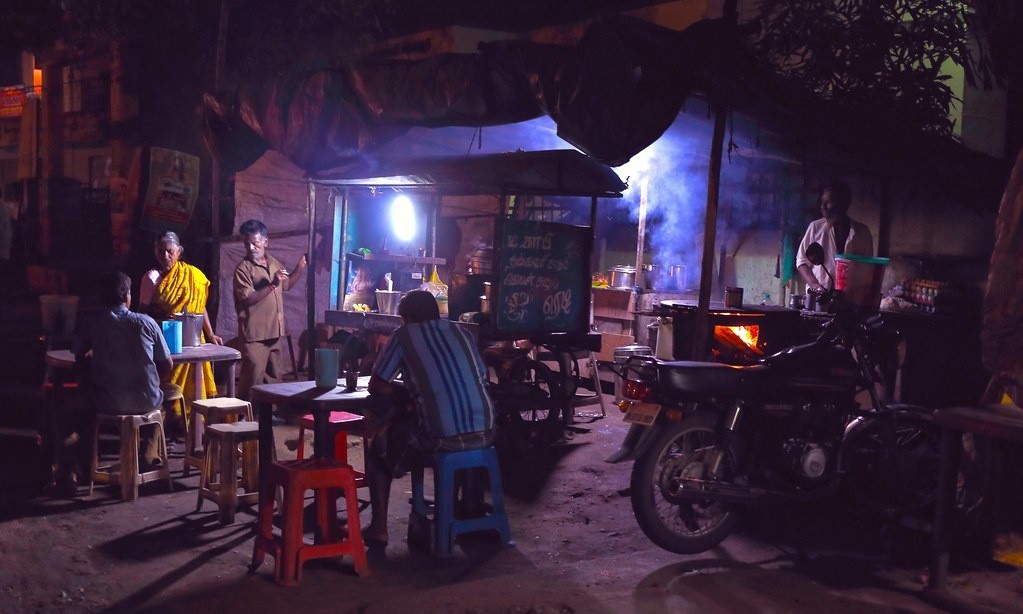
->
[604,242,947,555]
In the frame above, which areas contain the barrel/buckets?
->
[646,322,658,356]
[173,313,204,346]
[666,264,695,292]
[612,342,652,403]
[38,295,79,332]
[608,266,636,287]
[642,264,663,289]
[834,254,890,316]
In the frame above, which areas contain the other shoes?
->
[166,441,176,451]
[361,524,388,548]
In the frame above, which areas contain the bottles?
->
[761,294,773,306]
[356,248,370,254]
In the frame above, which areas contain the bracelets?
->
[268,283,277,291]
[207,333,214,336]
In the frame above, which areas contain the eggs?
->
[904,284,938,304]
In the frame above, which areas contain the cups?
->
[314,349,340,388]
[344,371,360,391]
[723,286,744,308]
[162,320,183,355]
[789,294,806,310]
[375,290,400,315]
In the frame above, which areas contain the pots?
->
[471,244,494,275]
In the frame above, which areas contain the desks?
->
[250,374,494,521]
[46,342,241,482]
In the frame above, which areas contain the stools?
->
[39,382,516,585]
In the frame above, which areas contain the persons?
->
[233,219,308,425]
[361,290,496,545]
[352,262,400,310]
[796,182,874,312]
[70,232,223,487]
